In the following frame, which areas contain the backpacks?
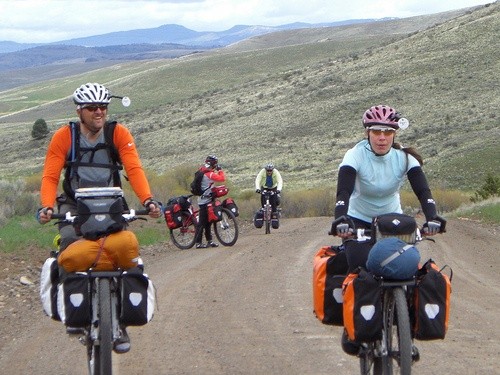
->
[164,198,183,229]
[190,169,214,196]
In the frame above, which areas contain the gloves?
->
[276,190,280,194]
[215,165,221,170]
[256,189,261,193]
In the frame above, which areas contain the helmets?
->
[205,155,218,164]
[362,105,402,131]
[264,162,274,170]
[73,83,111,104]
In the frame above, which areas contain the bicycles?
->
[256,190,280,234]
[170,191,239,250]
[50,207,150,375]
[327,226,447,375]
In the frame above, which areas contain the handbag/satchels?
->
[222,198,240,219]
[40,187,159,327]
[254,210,265,228]
[207,200,223,223]
[272,211,279,229]
[312,212,453,342]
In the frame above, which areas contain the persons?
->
[35,83,163,354]
[196,154,226,249]
[331,104,446,366]
[255,162,283,215]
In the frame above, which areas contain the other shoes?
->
[196,243,208,248]
[113,326,131,354]
[341,333,360,355]
[411,345,420,361]
[66,326,84,334]
[208,241,218,247]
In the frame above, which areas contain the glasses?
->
[369,128,394,135]
[80,103,108,112]
[267,170,272,172]
